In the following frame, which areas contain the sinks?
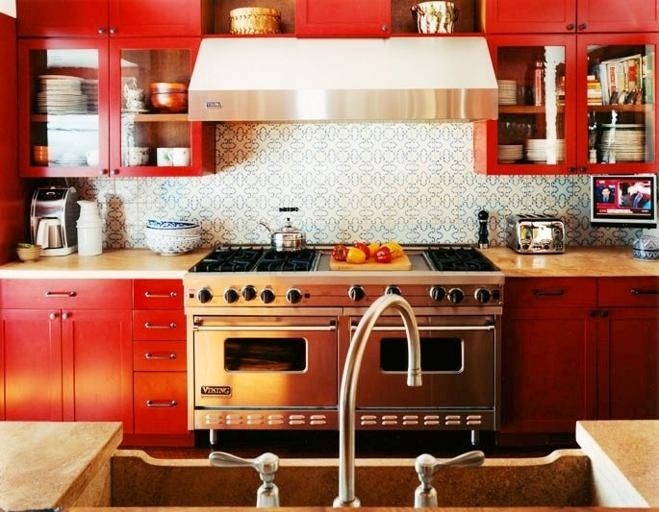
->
[69,449,650,512]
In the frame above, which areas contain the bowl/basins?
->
[229,6,283,35]
[16,243,41,262]
[33,146,53,166]
[121,77,148,113]
[150,82,186,112]
[123,146,149,165]
[411,1,460,34]
[144,219,202,256]
[632,236,659,260]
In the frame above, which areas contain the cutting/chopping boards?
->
[330,254,411,271]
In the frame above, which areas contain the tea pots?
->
[258,217,307,251]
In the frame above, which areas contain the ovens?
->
[182,280,505,428]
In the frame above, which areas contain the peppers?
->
[331,242,403,263]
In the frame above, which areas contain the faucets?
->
[333,294,424,508]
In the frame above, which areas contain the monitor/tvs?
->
[590,174,657,223]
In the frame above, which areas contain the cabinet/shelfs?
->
[502,276,659,448]
[474,1,659,175]
[201,1,482,37]
[132,280,195,451]
[15,0,216,177]
[0,277,132,448]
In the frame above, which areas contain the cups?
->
[157,148,190,166]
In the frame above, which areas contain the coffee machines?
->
[30,185,80,257]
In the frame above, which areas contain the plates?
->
[55,160,87,166]
[599,123,645,162]
[526,138,564,164]
[82,80,99,113]
[36,75,88,115]
[498,79,517,105]
[497,144,523,164]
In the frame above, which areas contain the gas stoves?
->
[422,244,500,271]
[188,244,322,271]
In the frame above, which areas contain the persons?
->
[597,187,614,203]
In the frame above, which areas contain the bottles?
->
[532,61,545,107]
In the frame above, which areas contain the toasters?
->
[505,214,566,254]
